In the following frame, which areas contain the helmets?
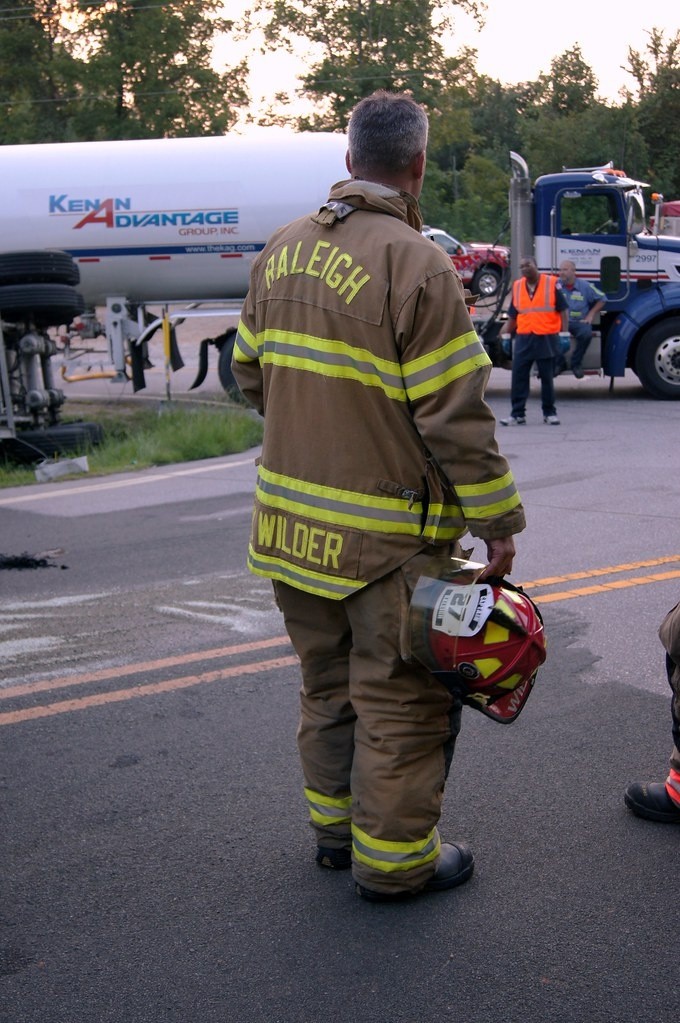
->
[411,565,548,722]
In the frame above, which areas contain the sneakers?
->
[500,417,526,425]
[544,417,559,424]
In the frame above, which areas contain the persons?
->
[624,599,680,824]
[227,91,606,906]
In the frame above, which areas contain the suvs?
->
[421,222,510,297]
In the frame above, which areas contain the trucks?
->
[0,132,680,413]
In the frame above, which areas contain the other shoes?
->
[315,847,352,871]
[553,363,567,378]
[355,841,474,904]
[573,364,584,378]
[624,781,680,824]
